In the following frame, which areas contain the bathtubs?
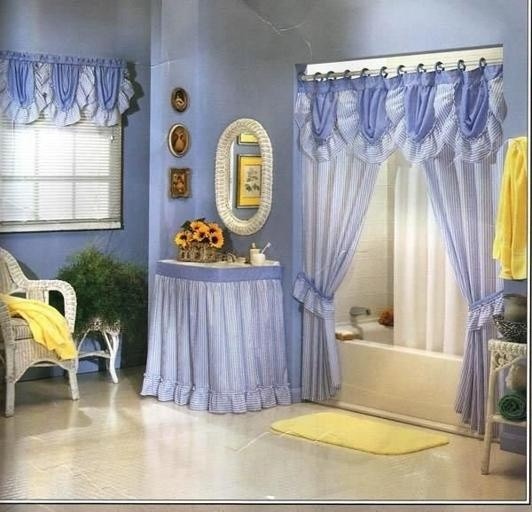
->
[313,322,496,441]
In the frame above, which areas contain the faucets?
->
[350,307,371,316]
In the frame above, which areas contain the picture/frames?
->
[236,154,262,209]
[172,88,187,112]
[237,133,259,145]
[170,168,191,198]
[168,124,189,158]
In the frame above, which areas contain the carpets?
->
[270,413,450,455]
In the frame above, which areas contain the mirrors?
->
[215,118,274,235]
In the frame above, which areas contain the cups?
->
[249,248,266,266]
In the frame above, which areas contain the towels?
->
[492,136,529,282]
[0,293,76,362]
[497,390,527,421]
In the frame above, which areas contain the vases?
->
[177,243,217,263]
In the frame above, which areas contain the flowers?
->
[174,217,225,249]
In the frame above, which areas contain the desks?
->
[480,339,527,476]
[141,259,290,414]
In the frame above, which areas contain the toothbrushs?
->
[261,242,271,254]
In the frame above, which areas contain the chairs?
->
[0,247,81,418]
